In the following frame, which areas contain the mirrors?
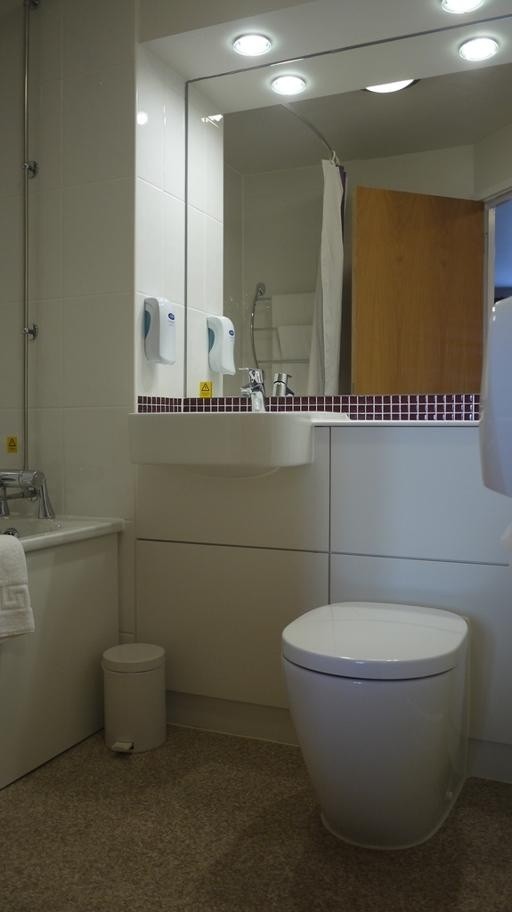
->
[184,15,512,398]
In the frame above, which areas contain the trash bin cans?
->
[102,642,168,755]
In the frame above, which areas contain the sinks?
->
[1,513,58,539]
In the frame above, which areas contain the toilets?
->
[280,601,471,843]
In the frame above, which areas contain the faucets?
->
[271,371,296,396]
[237,366,266,409]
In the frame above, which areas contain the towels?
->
[0,534,37,639]
